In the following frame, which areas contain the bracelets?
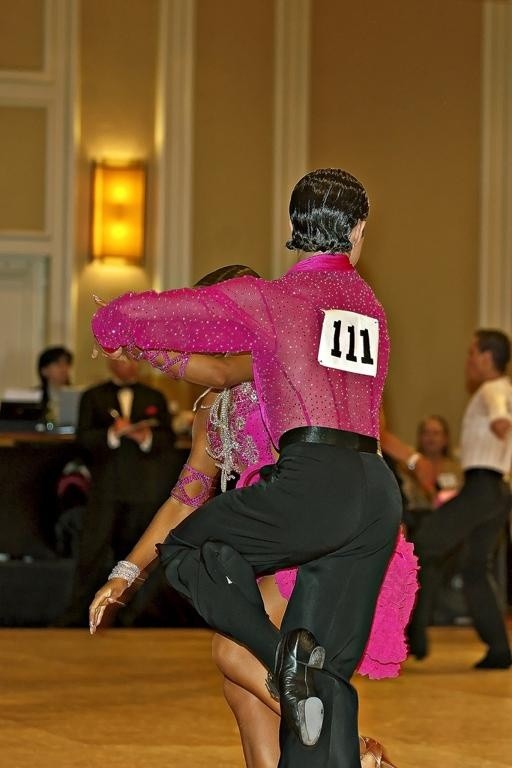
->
[107,557,141,589]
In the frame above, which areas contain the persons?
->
[47,350,192,627]
[87,266,424,768]
[90,165,402,768]
[36,345,74,425]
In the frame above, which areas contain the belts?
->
[279,426,378,454]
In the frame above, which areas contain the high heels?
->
[359,735,396,768]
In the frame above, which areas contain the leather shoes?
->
[266,627,325,748]
[474,657,511,669]
